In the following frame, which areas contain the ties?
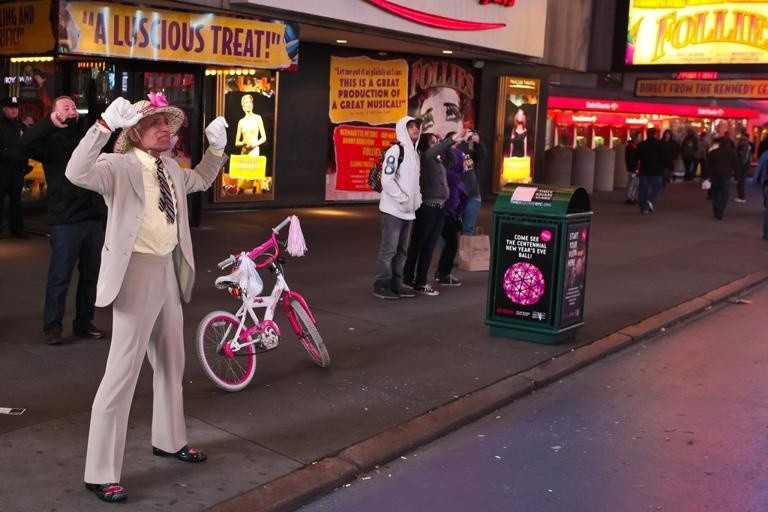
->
[155,157,175,225]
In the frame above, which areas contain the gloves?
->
[101,97,144,132]
[205,117,229,150]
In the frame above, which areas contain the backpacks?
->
[368,142,404,192]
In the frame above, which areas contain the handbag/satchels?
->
[229,150,267,180]
[456,226,491,271]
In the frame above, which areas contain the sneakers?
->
[732,198,746,203]
[439,274,461,286]
[153,446,206,462]
[435,271,459,282]
[46,332,62,345]
[641,209,649,215]
[73,327,105,339]
[85,483,128,502]
[413,284,440,296]
[402,280,415,289]
[646,199,656,214]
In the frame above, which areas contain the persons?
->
[411,84,466,141]
[370,115,488,300]
[509,108,529,157]
[17,96,109,347]
[0,96,34,239]
[623,125,768,242]
[64,89,230,502]
[234,94,267,156]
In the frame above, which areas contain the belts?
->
[422,201,446,209]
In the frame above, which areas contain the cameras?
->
[464,129,477,143]
[62,116,76,129]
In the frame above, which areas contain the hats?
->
[1,96,19,107]
[112,100,185,154]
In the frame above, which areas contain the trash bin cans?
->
[484,183,594,345]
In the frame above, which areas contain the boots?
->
[372,279,400,300]
[391,278,416,298]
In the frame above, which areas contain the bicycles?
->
[195,218,332,392]
